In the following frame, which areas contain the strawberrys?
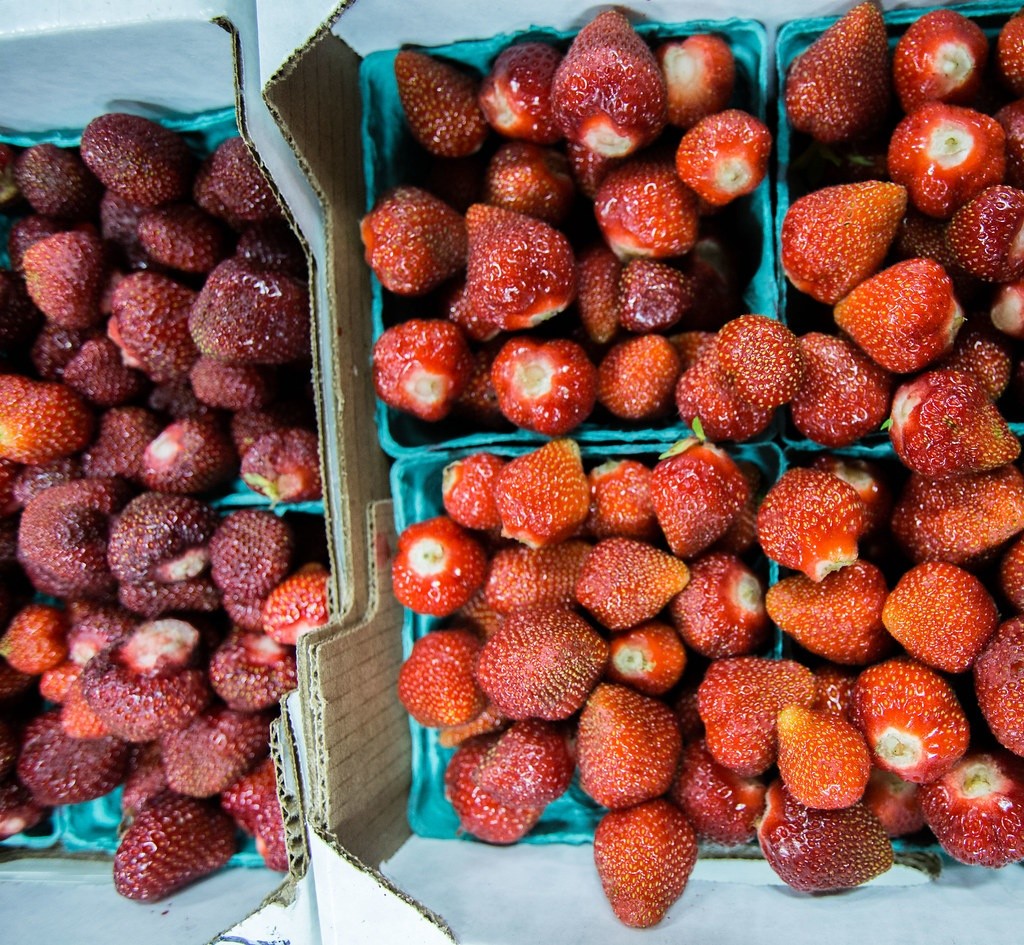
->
[0,111,334,905]
[357,0,1024,928]
